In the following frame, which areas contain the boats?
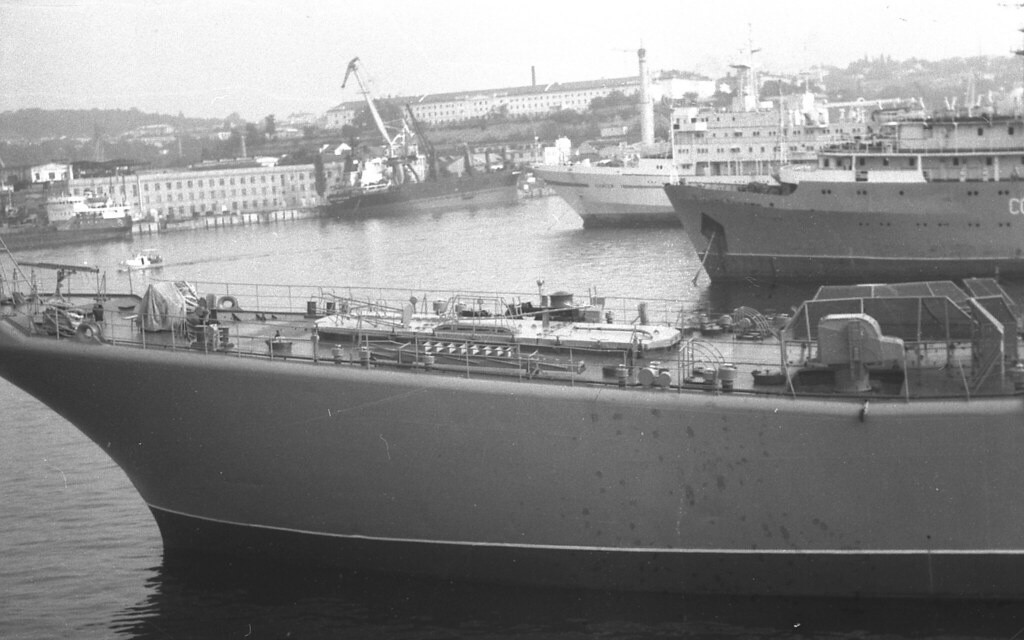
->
[533,44,930,233]
[662,103,1023,293]
[0,258,1024,614]
[315,57,523,223]
